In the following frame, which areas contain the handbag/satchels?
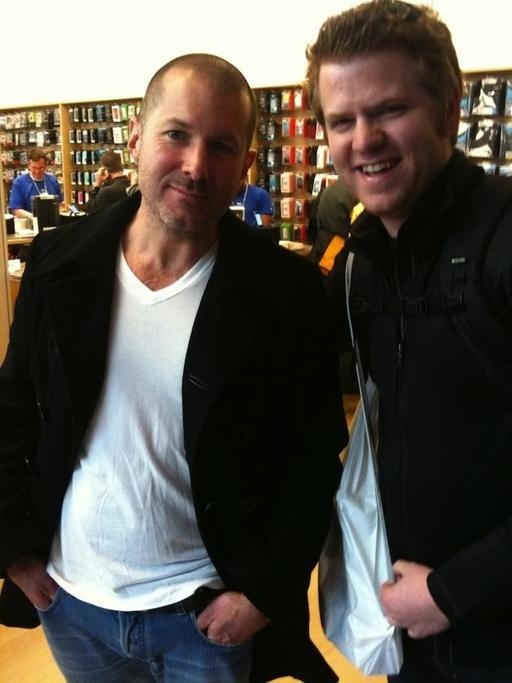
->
[318,251,406,677]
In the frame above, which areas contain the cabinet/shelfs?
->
[0,98,144,369]
[244,86,339,245]
[455,69,512,178]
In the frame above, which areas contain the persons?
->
[80,150,133,214]
[303,173,362,280]
[1,50,351,683]
[304,0,510,683]
[6,146,61,233]
[228,173,273,229]
